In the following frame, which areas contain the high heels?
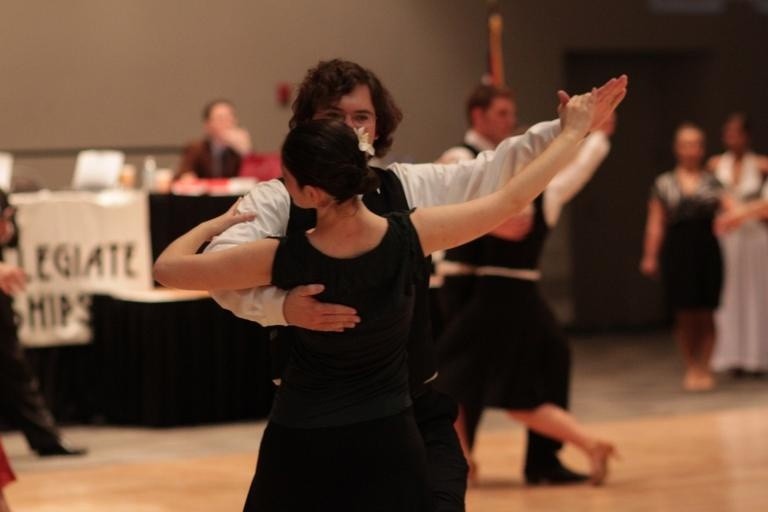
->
[590,438,621,486]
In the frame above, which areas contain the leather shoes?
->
[33,446,85,455]
[527,468,589,484]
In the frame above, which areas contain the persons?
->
[152,84,600,512]
[171,98,253,180]
[0,187,87,459]
[430,77,622,488]
[199,58,632,511]
[0,261,31,511]
[640,111,768,393]
